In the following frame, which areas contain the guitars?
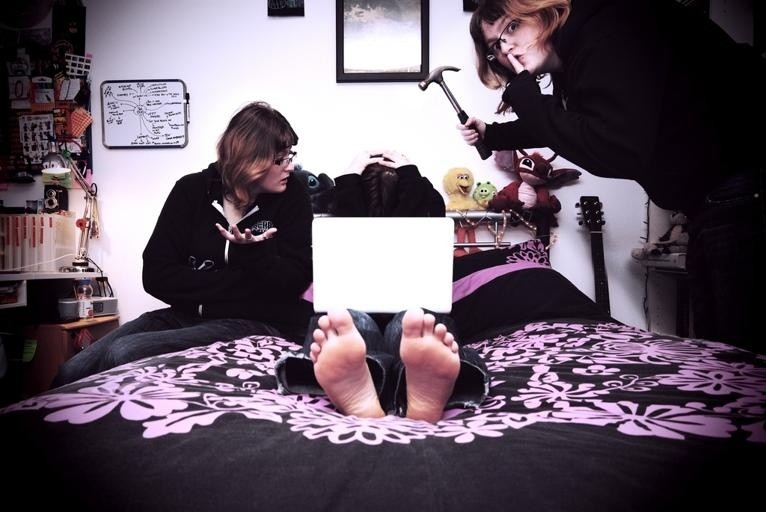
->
[576,196,611,316]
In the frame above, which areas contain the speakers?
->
[44,184,68,214]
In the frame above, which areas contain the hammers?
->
[417,64,492,160]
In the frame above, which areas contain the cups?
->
[57,298,77,321]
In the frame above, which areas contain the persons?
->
[275,163,489,426]
[458,0,766,358]
[50,102,314,385]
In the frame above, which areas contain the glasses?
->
[483,19,520,64]
[272,150,297,165]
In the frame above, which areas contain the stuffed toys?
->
[632,211,690,261]
[490,148,581,226]
[474,182,497,204]
[439,168,485,257]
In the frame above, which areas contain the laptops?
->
[312,215,455,314]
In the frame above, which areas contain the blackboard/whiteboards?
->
[99,79,190,149]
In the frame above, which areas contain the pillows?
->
[449,238,611,346]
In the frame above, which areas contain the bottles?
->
[77,280,94,322]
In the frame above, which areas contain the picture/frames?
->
[337,0,430,83]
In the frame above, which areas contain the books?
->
[0,211,76,273]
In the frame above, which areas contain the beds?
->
[0,238,763,505]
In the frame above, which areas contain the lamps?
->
[43,150,100,272]
